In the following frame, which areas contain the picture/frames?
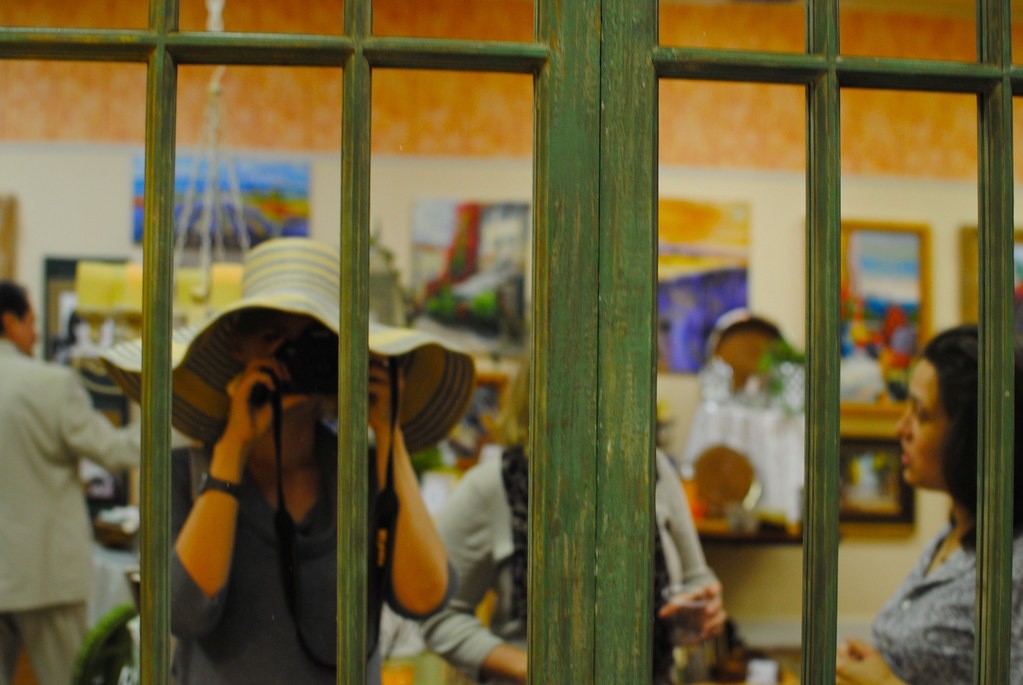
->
[841,219,935,356]
[43,256,136,509]
[957,224,1023,325]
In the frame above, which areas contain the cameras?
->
[254,325,340,403]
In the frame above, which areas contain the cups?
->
[670,598,712,684]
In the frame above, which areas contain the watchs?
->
[196,471,248,502]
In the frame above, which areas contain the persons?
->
[98,237,476,685]
[680,308,810,536]
[0,278,143,685]
[414,343,730,685]
[838,320,1023,685]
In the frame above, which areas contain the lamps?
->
[76,0,270,323]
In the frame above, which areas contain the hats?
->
[103,237,474,454]
